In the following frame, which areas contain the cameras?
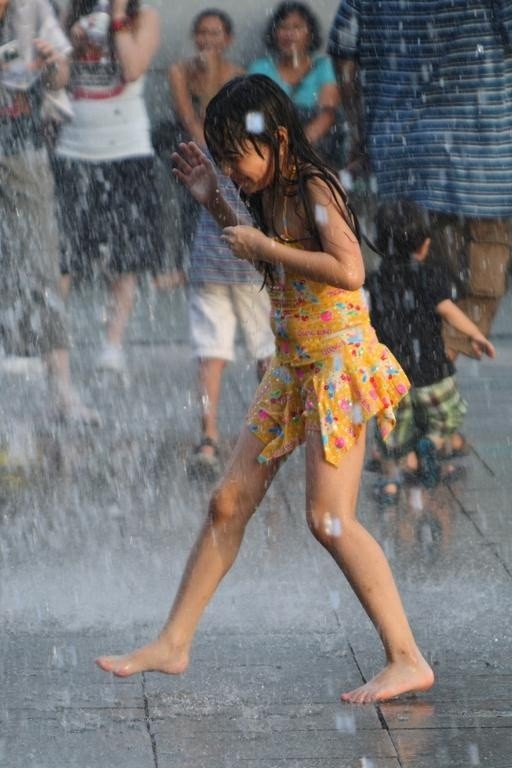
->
[0,38,23,66]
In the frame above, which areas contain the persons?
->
[0,0,510,502]
[95,69,437,705]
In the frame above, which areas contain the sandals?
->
[185,437,220,481]
[370,477,403,506]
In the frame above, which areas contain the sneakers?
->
[46,344,126,425]
[366,426,466,480]
